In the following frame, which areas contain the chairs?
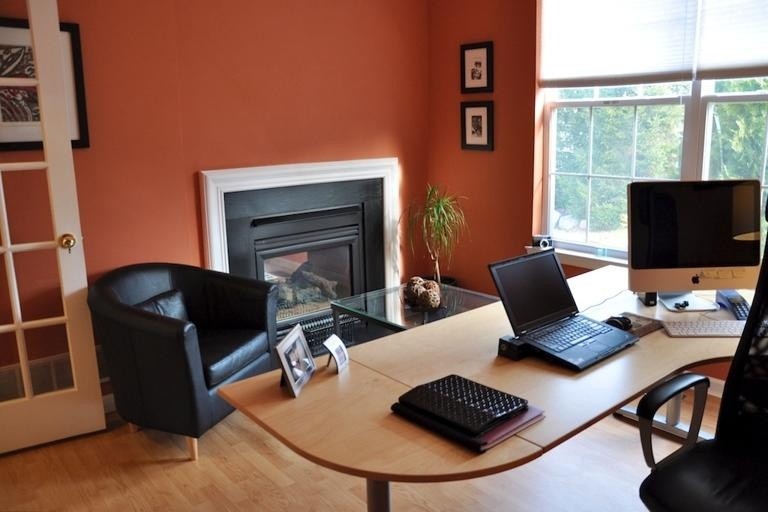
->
[637,199,768,512]
[87,263,280,461]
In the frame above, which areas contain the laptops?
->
[488,247,641,373]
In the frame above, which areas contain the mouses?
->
[606,316,633,331]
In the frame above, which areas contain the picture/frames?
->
[0,17,89,151]
[461,101,494,150]
[323,334,349,374]
[274,324,316,398]
[460,41,493,93]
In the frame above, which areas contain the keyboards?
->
[661,319,768,338]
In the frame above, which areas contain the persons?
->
[471,61,482,80]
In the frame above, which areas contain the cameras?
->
[532,235,552,249]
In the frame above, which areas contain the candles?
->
[399,182,470,287]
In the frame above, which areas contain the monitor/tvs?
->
[626,179,762,312]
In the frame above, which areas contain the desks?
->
[216,265,763,512]
